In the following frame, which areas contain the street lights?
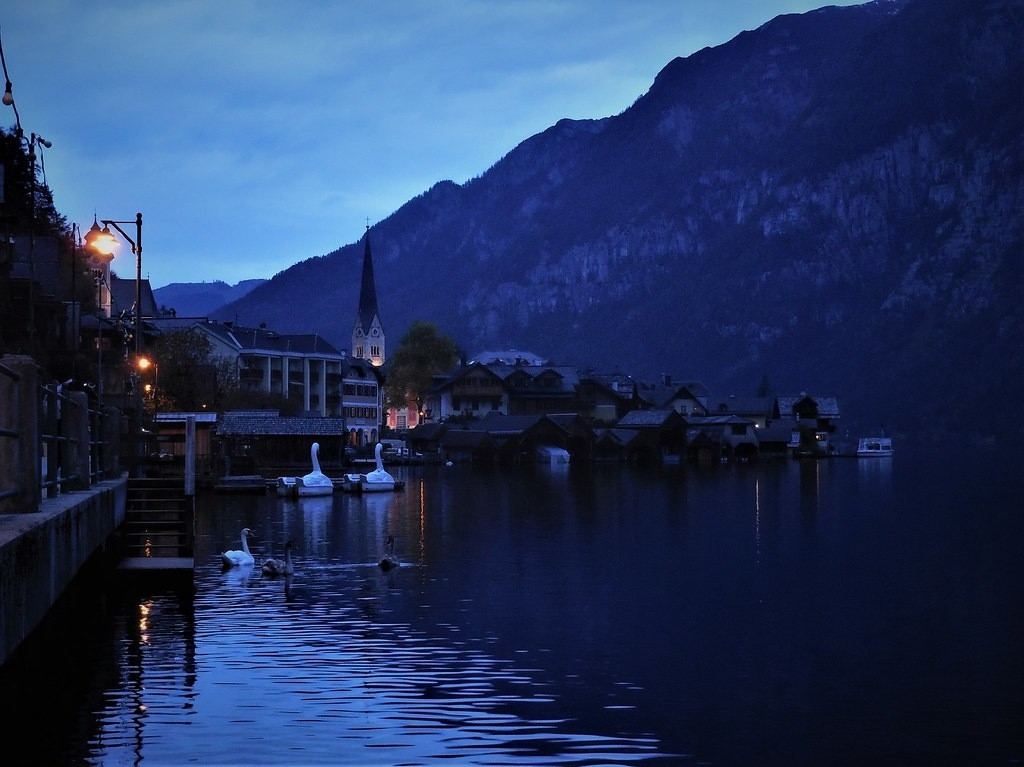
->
[92,211,158,429]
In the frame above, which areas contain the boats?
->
[213,476,267,493]
[343,441,395,492]
[276,441,334,497]
[856,437,895,457]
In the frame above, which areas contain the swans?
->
[219,528,256,567]
[379,535,402,569]
[260,541,298,577]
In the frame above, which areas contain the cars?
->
[384,448,397,459]
[396,447,423,458]
